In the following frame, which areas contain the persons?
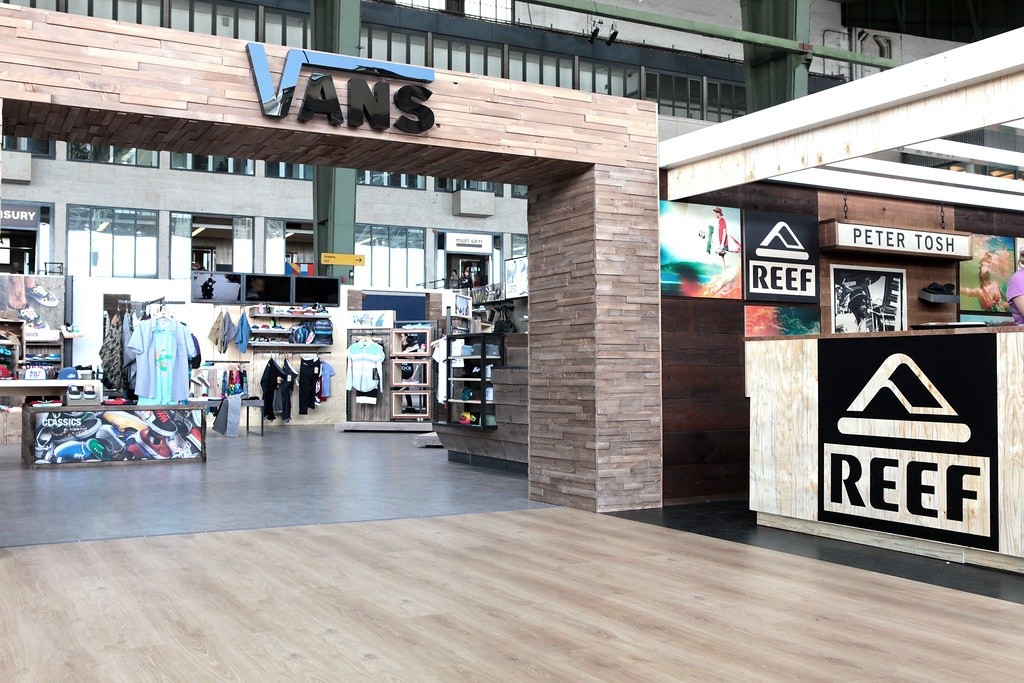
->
[449,265,474,288]
[401,334,418,411]
[960,266,1007,311]
[7,276,59,331]
[202,278,216,299]
[713,208,729,289]
[1005,249,1024,325]
[835,288,872,333]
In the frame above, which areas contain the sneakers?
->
[7,303,50,329]
[25,284,59,307]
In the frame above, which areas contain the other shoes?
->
[75,364,92,370]
[0,330,60,380]
[29,382,203,464]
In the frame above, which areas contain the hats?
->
[848,289,868,309]
[713,207,723,216]
[56,368,78,379]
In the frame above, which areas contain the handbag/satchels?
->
[461,387,474,400]
[493,305,516,333]
